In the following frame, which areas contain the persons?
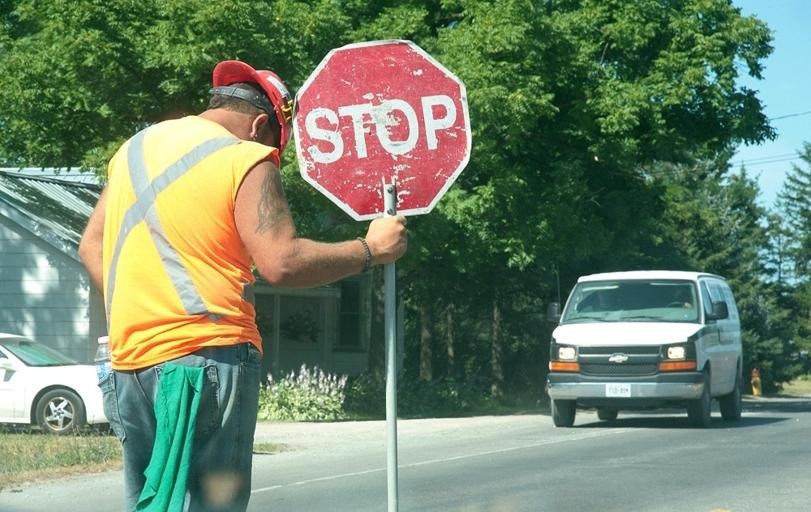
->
[77,59,408,509]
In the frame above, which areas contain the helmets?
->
[212,61,293,155]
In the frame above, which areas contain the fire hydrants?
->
[747,366,762,396]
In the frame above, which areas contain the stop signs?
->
[286,40,473,222]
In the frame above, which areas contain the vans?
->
[545,270,746,427]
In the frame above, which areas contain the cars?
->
[1,332,114,433]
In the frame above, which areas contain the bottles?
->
[94,335,113,384]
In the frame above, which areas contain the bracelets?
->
[358,237,372,274]
